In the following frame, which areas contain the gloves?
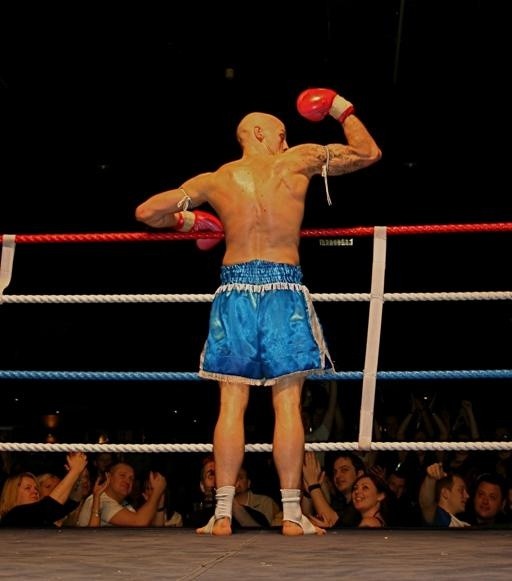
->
[296,89,355,124]
[173,211,225,251]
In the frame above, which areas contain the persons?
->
[1,388,511,534]
[134,85,384,538]
